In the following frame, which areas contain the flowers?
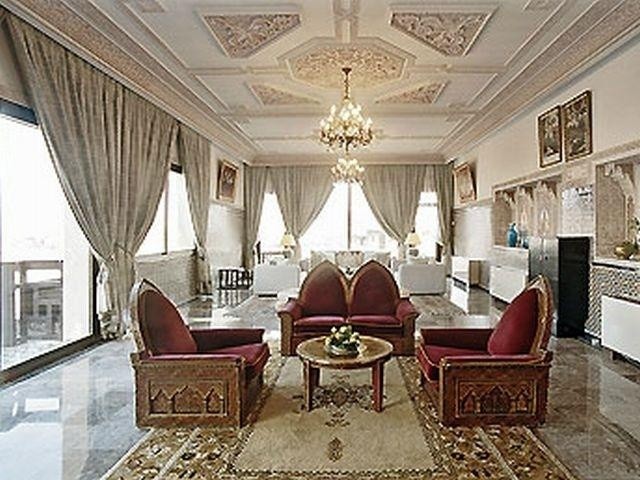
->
[324,323,363,352]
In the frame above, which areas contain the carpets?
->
[97,340,578,479]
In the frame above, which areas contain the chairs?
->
[127,278,271,428]
[417,272,556,427]
[253,250,447,296]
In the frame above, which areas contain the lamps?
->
[313,67,375,185]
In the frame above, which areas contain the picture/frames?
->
[538,90,592,168]
[453,161,476,203]
[218,160,238,201]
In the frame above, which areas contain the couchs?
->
[277,259,420,357]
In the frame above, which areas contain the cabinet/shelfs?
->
[529,238,589,337]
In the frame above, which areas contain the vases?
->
[329,345,358,358]
[507,222,518,247]
